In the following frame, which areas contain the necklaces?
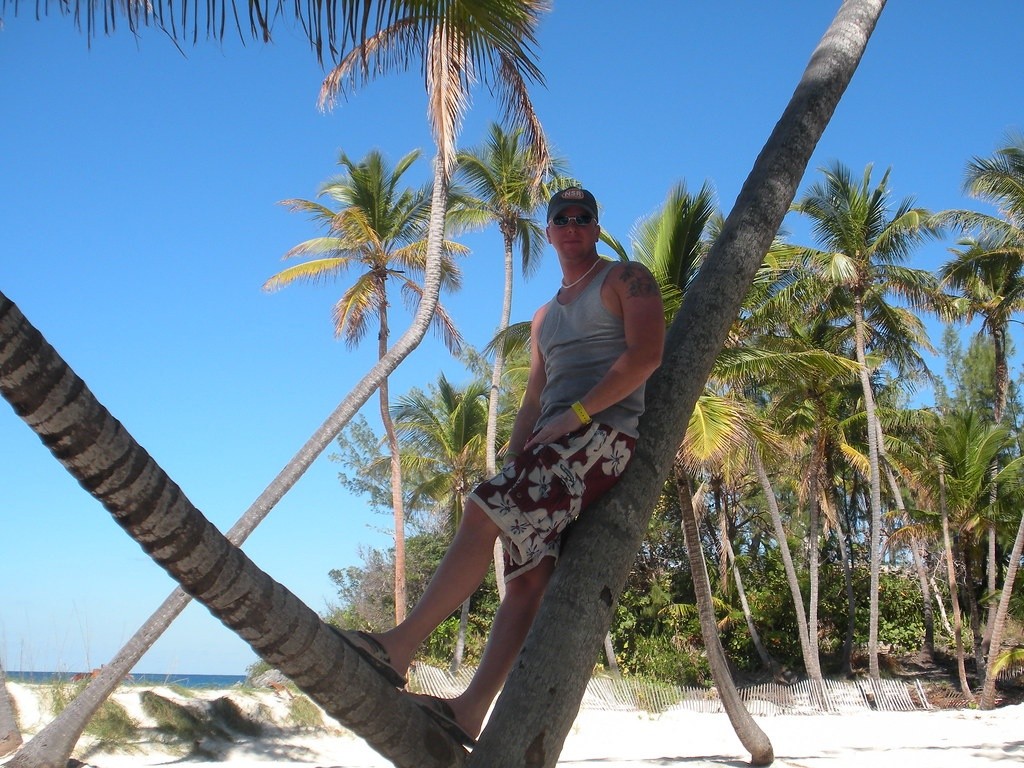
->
[557,256,603,289]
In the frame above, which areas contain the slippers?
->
[326,622,406,686]
[404,690,479,748]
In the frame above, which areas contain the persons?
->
[324,185,665,746]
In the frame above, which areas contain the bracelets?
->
[502,452,519,460]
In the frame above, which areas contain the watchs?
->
[571,399,592,427]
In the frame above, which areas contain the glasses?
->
[548,213,597,227]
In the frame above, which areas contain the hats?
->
[547,186,598,224]
